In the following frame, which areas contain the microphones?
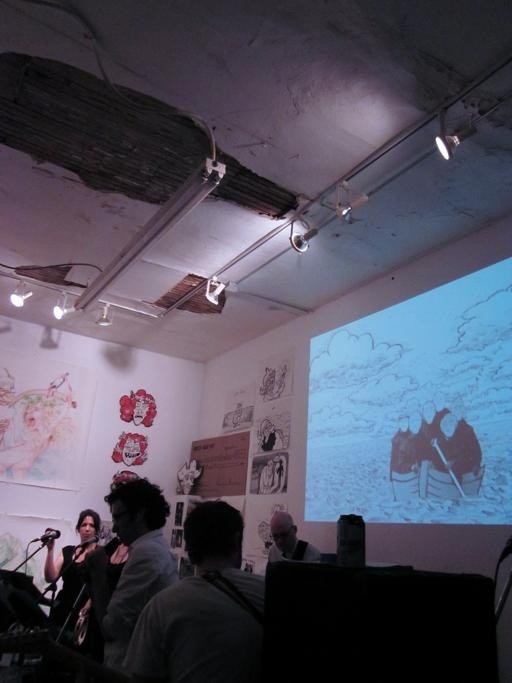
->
[33,530,60,542]
[75,535,99,548]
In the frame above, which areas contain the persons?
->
[76,537,129,663]
[122,501,267,682]
[94,480,184,677]
[267,511,320,562]
[45,509,104,638]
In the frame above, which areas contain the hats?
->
[270,510,294,533]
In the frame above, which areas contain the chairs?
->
[260,560,502,682]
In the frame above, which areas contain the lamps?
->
[50,293,76,320]
[203,275,226,307]
[336,180,369,224]
[73,156,229,315]
[9,278,35,309]
[93,305,113,325]
[290,215,317,254]
[435,113,477,160]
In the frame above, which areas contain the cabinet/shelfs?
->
[173,494,205,581]
